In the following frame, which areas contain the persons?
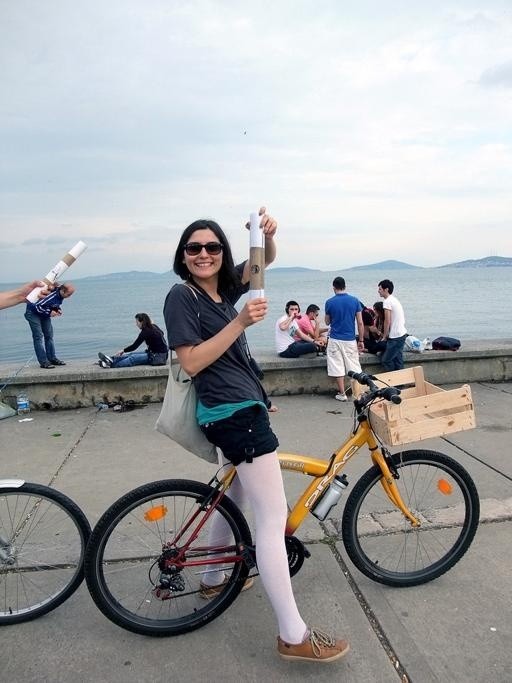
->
[297,304,328,343]
[324,276,364,402]
[361,302,387,356]
[162,206,351,663]
[0,280,60,311]
[275,301,324,358]
[97,313,168,368]
[24,282,75,369]
[378,279,407,371]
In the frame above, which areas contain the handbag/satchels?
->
[155,369,219,464]
[150,353,167,366]
[432,337,461,351]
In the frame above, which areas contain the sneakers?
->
[41,359,65,368]
[98,353,113,368]
[336,395,347,401]
[200,575,254,598]
[277,627,349,663]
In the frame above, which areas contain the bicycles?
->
[79,368,485,639]
[0,478,93,626]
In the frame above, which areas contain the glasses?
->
[184,242,223,256]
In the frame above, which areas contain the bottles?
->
[308,471,349,522]
[295,313,302,319]
[316,344,323,356]
[16,389,31,416]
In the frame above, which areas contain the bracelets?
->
[313,340,316,343]
[358,341,364,342]
